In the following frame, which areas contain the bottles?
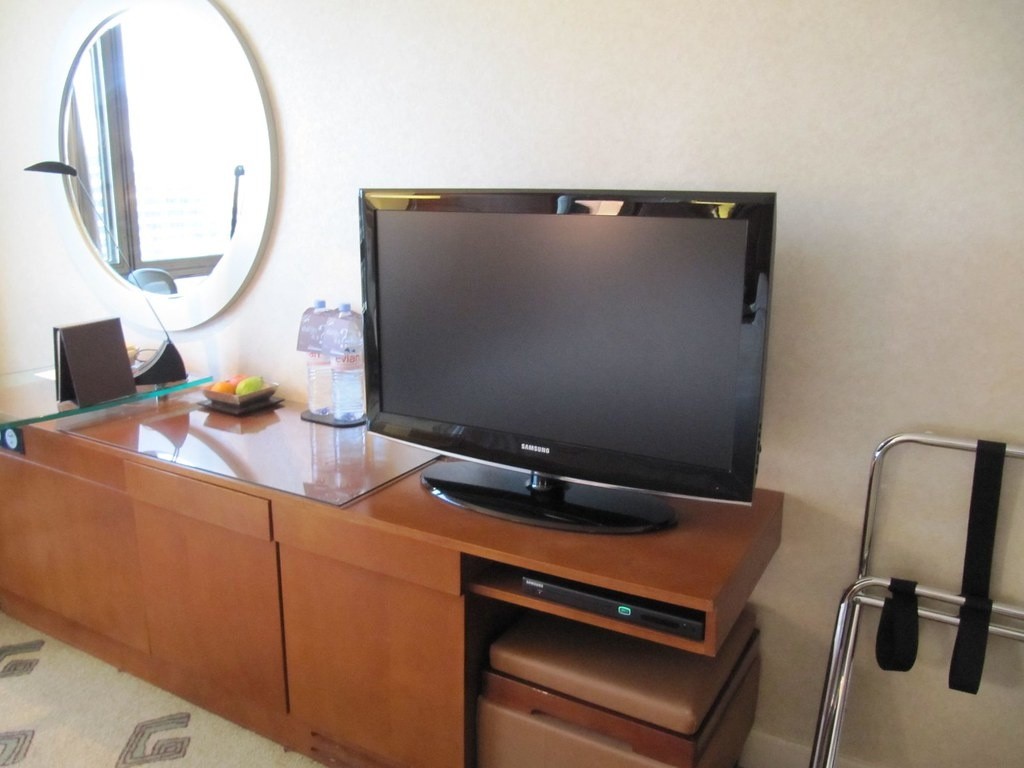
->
[329,305,362,420]
[307,301,332,413]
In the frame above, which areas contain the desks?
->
[2,359,790,768]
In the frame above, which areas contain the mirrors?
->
[55,0,281,336]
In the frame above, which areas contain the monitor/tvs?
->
[354,186,780,536]
